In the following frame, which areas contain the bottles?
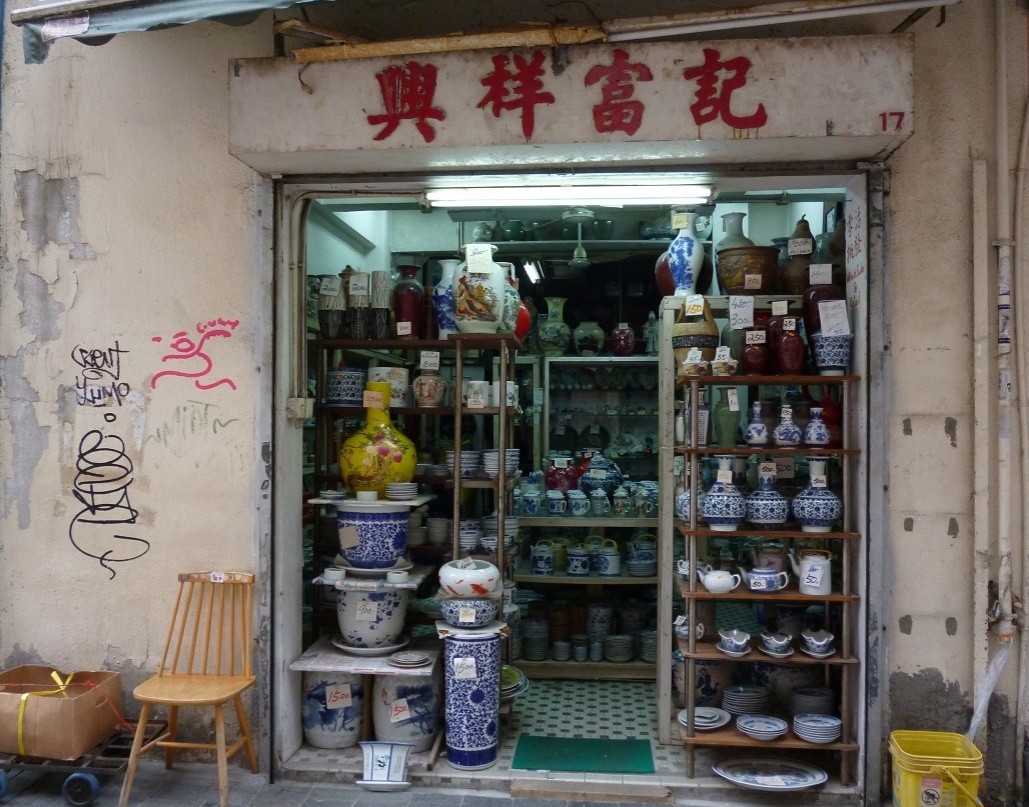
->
[413,365,446,407]
[472,220,585,241]
[317,242,657,357]
[339,381,416,499]
[299,633,500,769]
[655,211,844,533]
[675,598,739,707]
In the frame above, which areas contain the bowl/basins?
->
[446,449,520,479]
[337,504,410,647]
[718,628,835,653]
[408,514,520,628]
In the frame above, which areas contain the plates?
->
[551,366,657,389]
[677,646,842,792]
[549,423,611,451]
[625,560,657,577]
[319,489,347,499]
[500,589,657,707]
[332,562,433,668]
[385,463,448,501]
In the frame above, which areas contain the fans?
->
[538,210,638,280]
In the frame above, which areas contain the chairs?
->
[116,569,257,806]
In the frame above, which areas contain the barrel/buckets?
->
[887,729,985,807]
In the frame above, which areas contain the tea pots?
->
[675,536,833,639]
[528,533,621,577]
[626,533,658,560]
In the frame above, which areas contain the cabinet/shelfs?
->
[543,359,657,480]
[314,333,524,665]
[506,517,662,683]
[656,298,869,780]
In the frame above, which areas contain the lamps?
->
[422,184,718,208]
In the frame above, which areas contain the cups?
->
[449,379,519,408]
[512,480,659,518]
[591,219,614,240]
[326,366,410,408]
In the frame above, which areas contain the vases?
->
[340,382,418,501]
[677,296,854,529]
[549,455,624,497]
[473,218,614,240]
[303,672,366,749]
[336,505,410,569]
[412,365,445,408]
[362,741,415,783]
[322,243,654,356]
[640,212,847,298]
[373,675,443,753]
[333,588,407,648]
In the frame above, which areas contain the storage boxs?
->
[0,663,122,762]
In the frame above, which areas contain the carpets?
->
[512,735,654,773]
